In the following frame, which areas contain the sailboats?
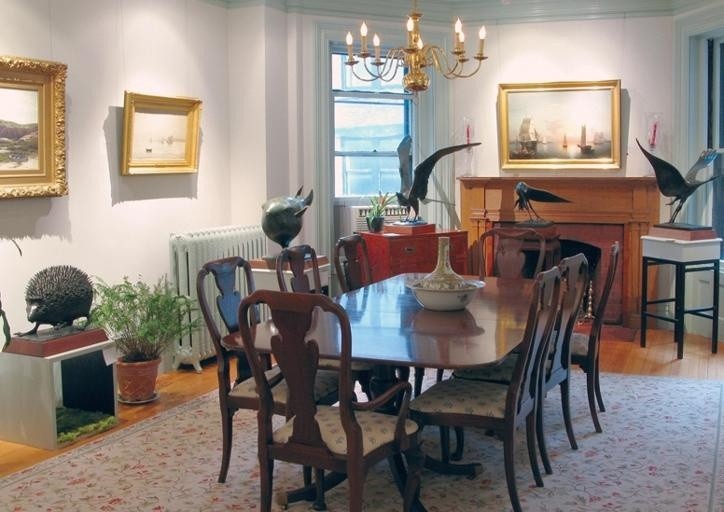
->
[508,118,537,158]
[578,124,592,153]
[563,136,567,148]
[593,132,604,145]
[542,137,548,144]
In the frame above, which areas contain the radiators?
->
[169,225,268,374]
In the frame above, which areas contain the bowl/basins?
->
[406,279,485,312]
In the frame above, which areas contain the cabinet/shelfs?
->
[352,231,468,287]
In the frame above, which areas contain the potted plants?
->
[366,190,398,233]
[76,272,207,404]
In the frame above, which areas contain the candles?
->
[462,117,471,145]
[649,121,657,144]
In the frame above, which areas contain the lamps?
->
[344,0,488,96]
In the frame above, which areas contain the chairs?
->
[238,290,420,512]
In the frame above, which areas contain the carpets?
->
[575,321,636,342]
[1,361,724,511]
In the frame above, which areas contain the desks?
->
[220,271,583,512]
[250,261,332,289]
[0,325,119,452]
[639,236,724,359]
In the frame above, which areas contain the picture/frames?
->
[122,90,203,176]
[0,55,69,201]
[497,78,621,171]
